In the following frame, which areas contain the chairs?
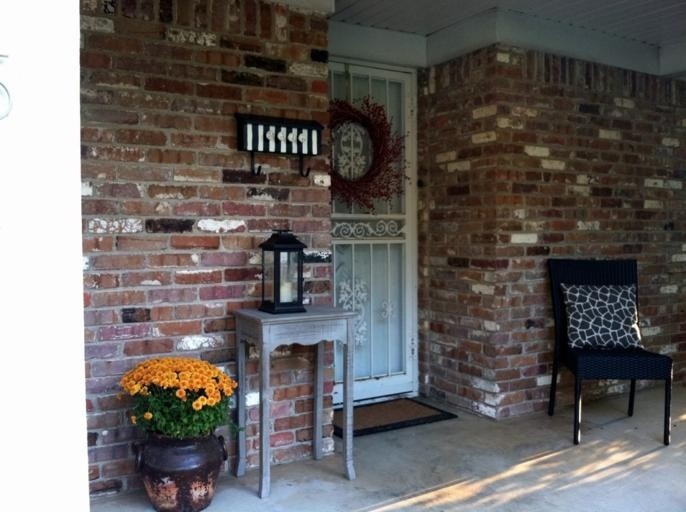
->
[546,258,674,445]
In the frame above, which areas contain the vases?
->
[132,433,228,510]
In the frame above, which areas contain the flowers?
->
[118,356,238,438]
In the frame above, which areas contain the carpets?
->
[332,396,458,439]
